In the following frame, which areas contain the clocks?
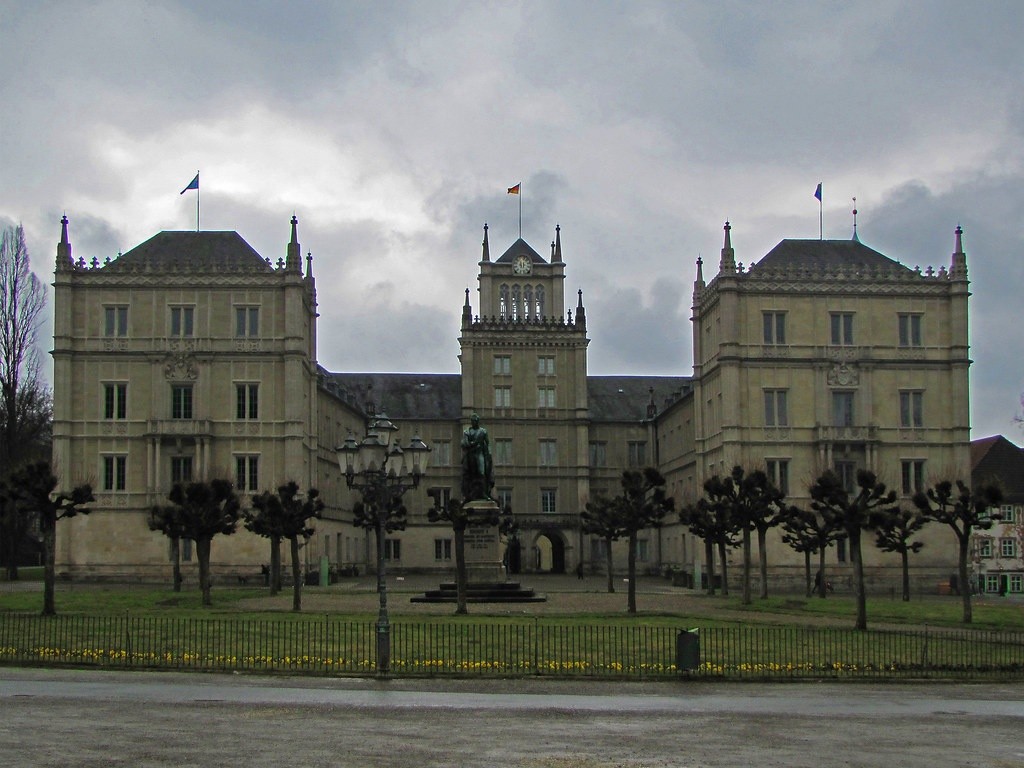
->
[514,256,531,274]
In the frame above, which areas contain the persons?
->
[948,569,959,595]
[459,414,494,501]
[260,564,269,586]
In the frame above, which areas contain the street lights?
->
[336,414,431,676]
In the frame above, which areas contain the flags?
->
[507,184,519,195]
[814,184,822,201]
[179,173,198,195]
